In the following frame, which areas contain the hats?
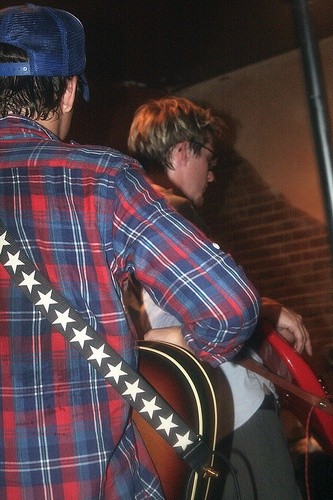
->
[1,3,89,102]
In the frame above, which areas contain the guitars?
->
[128,340,221,500]
[248,312,333,458]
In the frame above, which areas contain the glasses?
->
[191,143,218,169]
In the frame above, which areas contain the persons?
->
[117,97,315,500]
[0,0,261,500]
